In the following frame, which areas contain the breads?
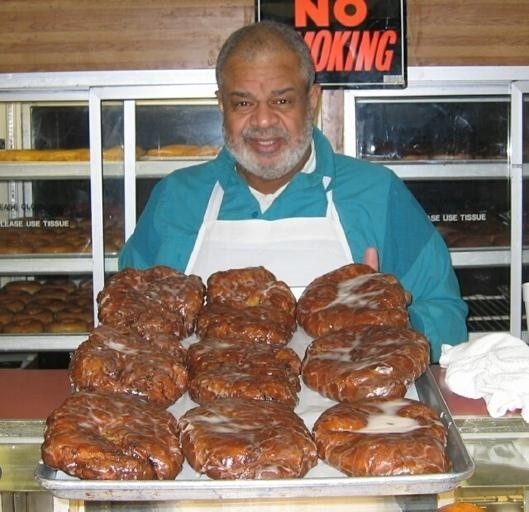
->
[0,262,453,481]
[431,210,512,250]
[0,142,224,163]
[358,133,507,163]
[1,220,123,260]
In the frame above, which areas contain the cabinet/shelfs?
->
[0,78,529,355]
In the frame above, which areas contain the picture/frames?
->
[251,1,410,91]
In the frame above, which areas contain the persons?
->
[116,18,470,364]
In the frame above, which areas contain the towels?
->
[426,328,529,428]
